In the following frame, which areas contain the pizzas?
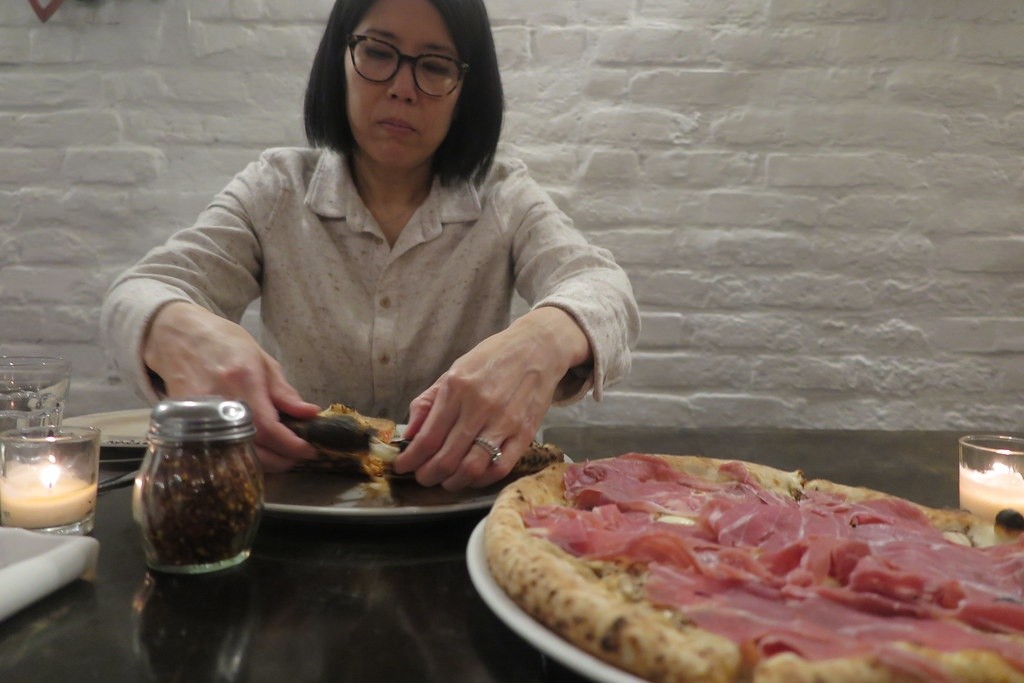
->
[485,451,1024,683]
[280,404,399,478]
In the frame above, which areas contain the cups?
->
[958,434,1024,522]
[0,355,71,434]
[0,425,102,535]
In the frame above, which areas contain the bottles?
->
[131,393,265,575]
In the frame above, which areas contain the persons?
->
[99,0,642,491]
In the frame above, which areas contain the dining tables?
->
[0,427,1024,683]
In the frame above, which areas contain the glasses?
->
[346,34,472,98]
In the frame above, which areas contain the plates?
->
[262,495,499,516]
[463,517,647,683]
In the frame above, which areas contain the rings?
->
[474,437,502,464]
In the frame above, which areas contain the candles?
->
[958,460,1024,521]
[0,459,97,530]
